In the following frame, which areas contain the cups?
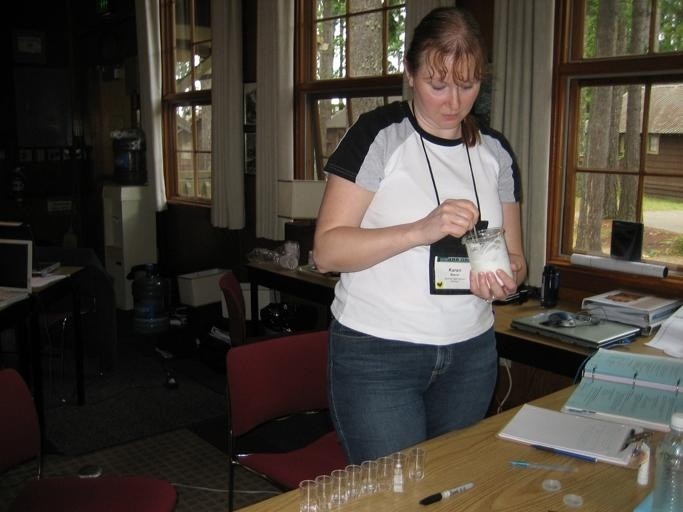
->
[460,226,513,289]
[299,445,425,511]
[247,241,316,271]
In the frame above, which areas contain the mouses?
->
[548,311,576,327]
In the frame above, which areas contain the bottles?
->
[539,263,560,308]
[650,412,683,511]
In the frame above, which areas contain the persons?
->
[313,6,527,466]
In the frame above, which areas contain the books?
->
[582,287,682,337]
[495,403,647,470]
[562,348,682,433]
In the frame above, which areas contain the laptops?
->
[511,308,641,351]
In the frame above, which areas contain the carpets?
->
[9,322,225,459]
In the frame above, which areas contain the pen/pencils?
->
[419,482,475,505]
[508,461,575,473]
[530,445,598,464]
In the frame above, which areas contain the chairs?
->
[227,329,350,511]
[0,363,179,512]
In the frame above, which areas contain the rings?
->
[482,294,495,304]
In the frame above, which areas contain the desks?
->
[0,265,86,455]
[237,252,683,512]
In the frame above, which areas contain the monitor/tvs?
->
[0,239,33,294]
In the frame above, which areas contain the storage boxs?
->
[176,268,233,309]
[217,282,280,322]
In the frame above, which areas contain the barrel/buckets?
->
[131,264,171,334]
[113,128,147,185]
